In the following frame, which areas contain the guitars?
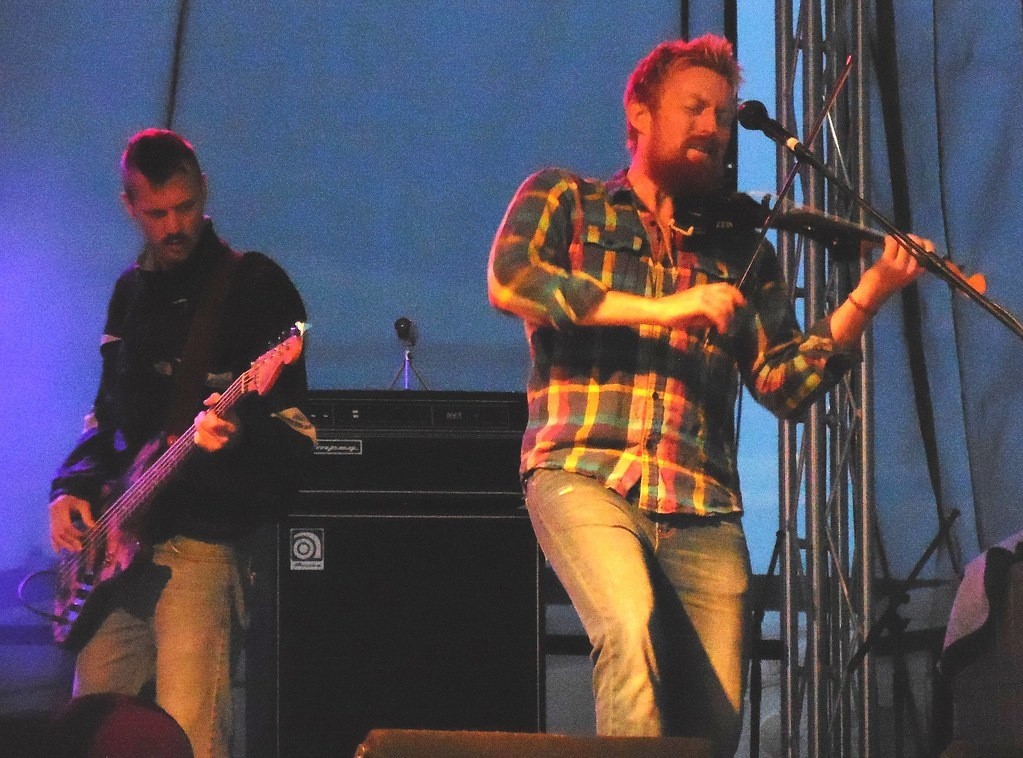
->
[52,320,313,642]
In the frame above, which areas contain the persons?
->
[48,128,317,758]
[487,31,985,758]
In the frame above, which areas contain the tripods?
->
[390,344,428,390]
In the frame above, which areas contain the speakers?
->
[246,489,546,758]
[353,728,719,758]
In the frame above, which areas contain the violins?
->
[672,182,987,299]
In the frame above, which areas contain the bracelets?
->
[848,293,878,315]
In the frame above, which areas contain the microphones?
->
[737,100,802,152]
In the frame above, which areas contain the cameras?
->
[395,318,419,346]
